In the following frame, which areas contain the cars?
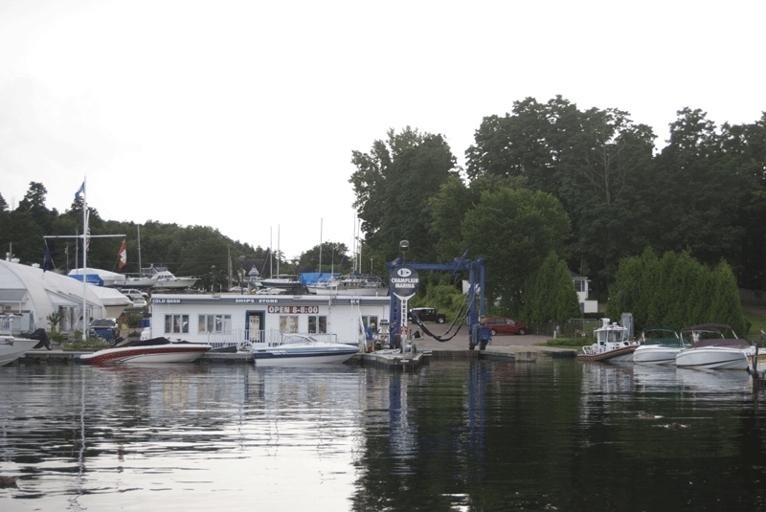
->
[90,318,120,339]
[479,316,528,335]
[229,286,270,294]
[120,288,149,310]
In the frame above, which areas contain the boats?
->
[576,318,765,379]
[253,333,360,364]
[0,336,41,365]
[140,264,202,289]
[80,337,211,363]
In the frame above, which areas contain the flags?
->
[41,239,56,272]
[114,239,129,269]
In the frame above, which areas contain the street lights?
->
[399,240,409,335]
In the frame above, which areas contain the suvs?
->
[410,307,447,324]
